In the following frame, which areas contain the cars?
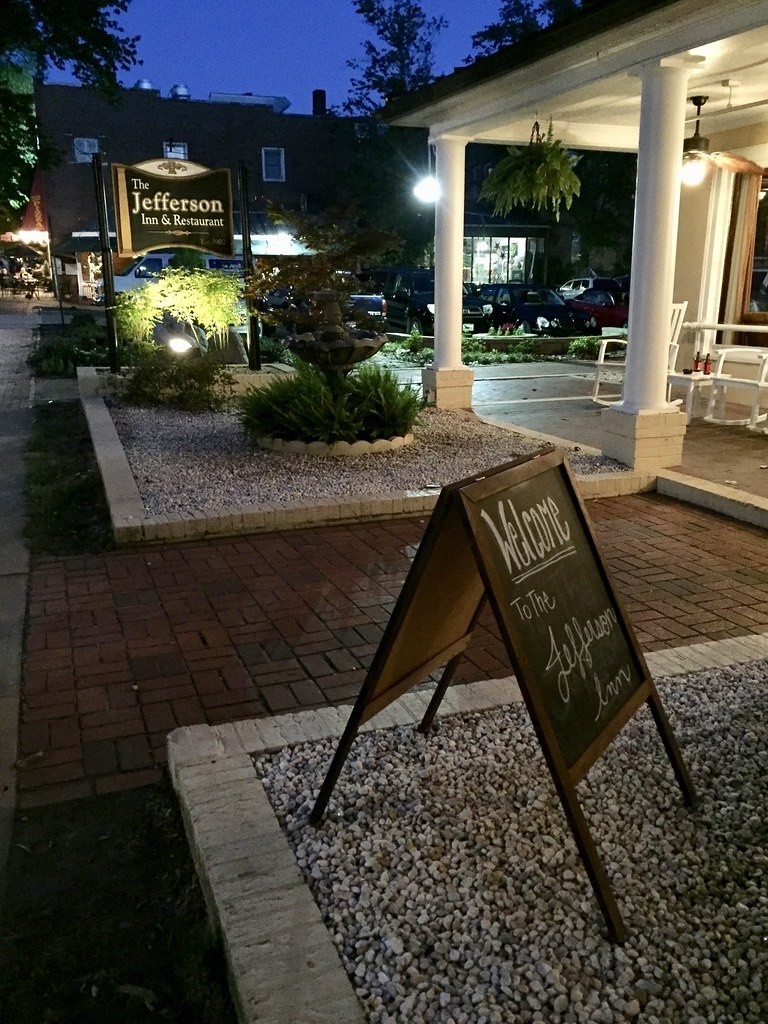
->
[95,248,389,336]
[555,277,624,302]
[475,282,582,338]
[564,283,629,329]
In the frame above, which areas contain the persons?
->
[0,252,104,298]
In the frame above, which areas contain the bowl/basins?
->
[683,368,692,374]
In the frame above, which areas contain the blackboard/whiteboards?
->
[350,443,654,796]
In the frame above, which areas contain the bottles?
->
[703,354,712,374]
[694,351,701,372]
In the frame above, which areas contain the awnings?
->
[0,231,343,257]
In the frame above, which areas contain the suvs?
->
[367,265,499,338]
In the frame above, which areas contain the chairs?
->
[592,300,688,407]
[503,294,510,303]
[704,347,768,434]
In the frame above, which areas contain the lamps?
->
[682,95,709,186]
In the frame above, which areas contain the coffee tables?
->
[666,371,731,420]
